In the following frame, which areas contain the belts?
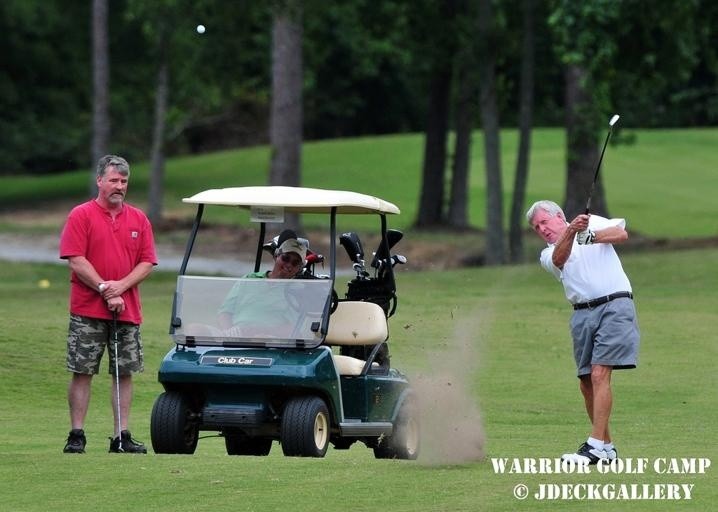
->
[573,291,633,310]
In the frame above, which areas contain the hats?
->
[278,238,308,264]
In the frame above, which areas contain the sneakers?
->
[108,430,147,453]
[561,442,618,465]
[63,429,86,453]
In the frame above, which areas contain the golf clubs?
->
[112,308,128,453]
[263,230,324,277]
[340,228,407,280]
[579,113,621,245]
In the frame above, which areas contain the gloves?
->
[576,229,595,246]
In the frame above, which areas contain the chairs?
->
[323,300,388,378]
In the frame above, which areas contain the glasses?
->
[281,255,299,266]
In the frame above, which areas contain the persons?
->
[526,199,642,466]
[181,237,310,354]
[57,155,162,454]
